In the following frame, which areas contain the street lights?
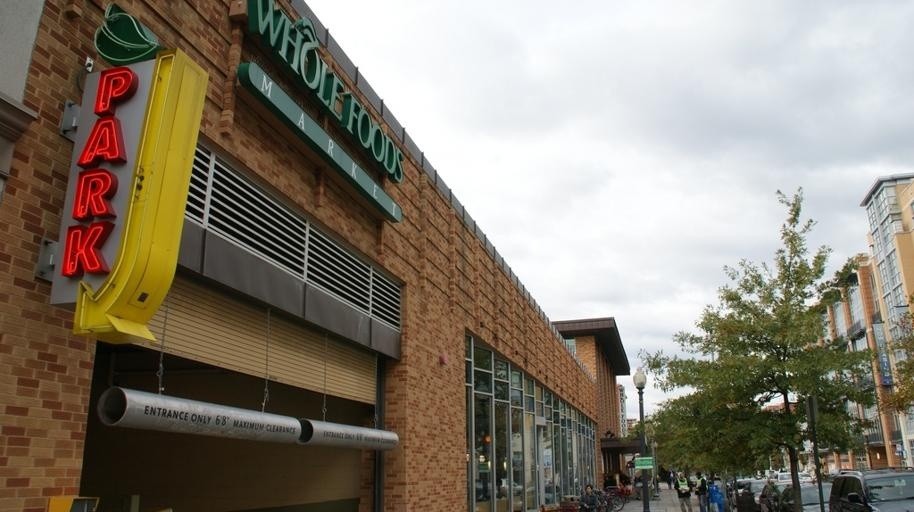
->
[633,366,654,512]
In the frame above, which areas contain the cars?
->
[472,474,558,501]
[690,469,914,512]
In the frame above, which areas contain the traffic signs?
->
[634,459,653,468]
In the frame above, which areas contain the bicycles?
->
[596,490,625,512]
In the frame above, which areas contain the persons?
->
[693,472,709,511]
[666,474,672,489]
[673,470,693,511]
[579,483,600,511]
[756,479,794,512]
